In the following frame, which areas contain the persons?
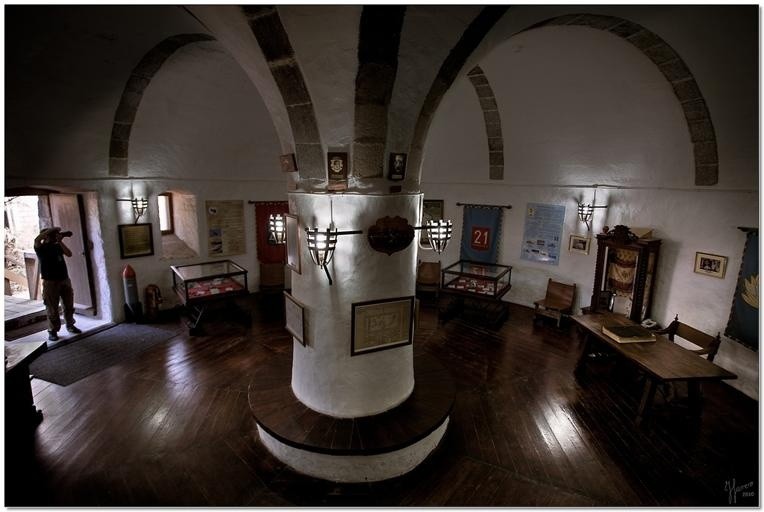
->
[575,240,584,250]
[702,259,712,270]
[33,227,81,342]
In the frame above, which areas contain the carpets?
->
[29,321,181,387]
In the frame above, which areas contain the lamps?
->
[409,219,453,255]
[577,201,608,231]
[269,214,286,247]
[305,226,362,285]
[131,197,148,224]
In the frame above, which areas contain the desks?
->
[571,310,737,417]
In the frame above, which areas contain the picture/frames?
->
[568,234,591,256]
[695,251,728,280]
[119,222,154,260]
[350,296,415,357]
[283,291,305,347]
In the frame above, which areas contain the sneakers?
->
[49,333,58,341]
[67,326,81,333]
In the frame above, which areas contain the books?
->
[600,324,656,345]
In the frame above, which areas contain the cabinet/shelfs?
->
[170,260,251,337]
[443,259,512,331]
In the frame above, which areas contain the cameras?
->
[49,229,73,242]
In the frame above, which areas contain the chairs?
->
[533,278,577,337]
[652,314,722,362]
[417,260,442,303]
